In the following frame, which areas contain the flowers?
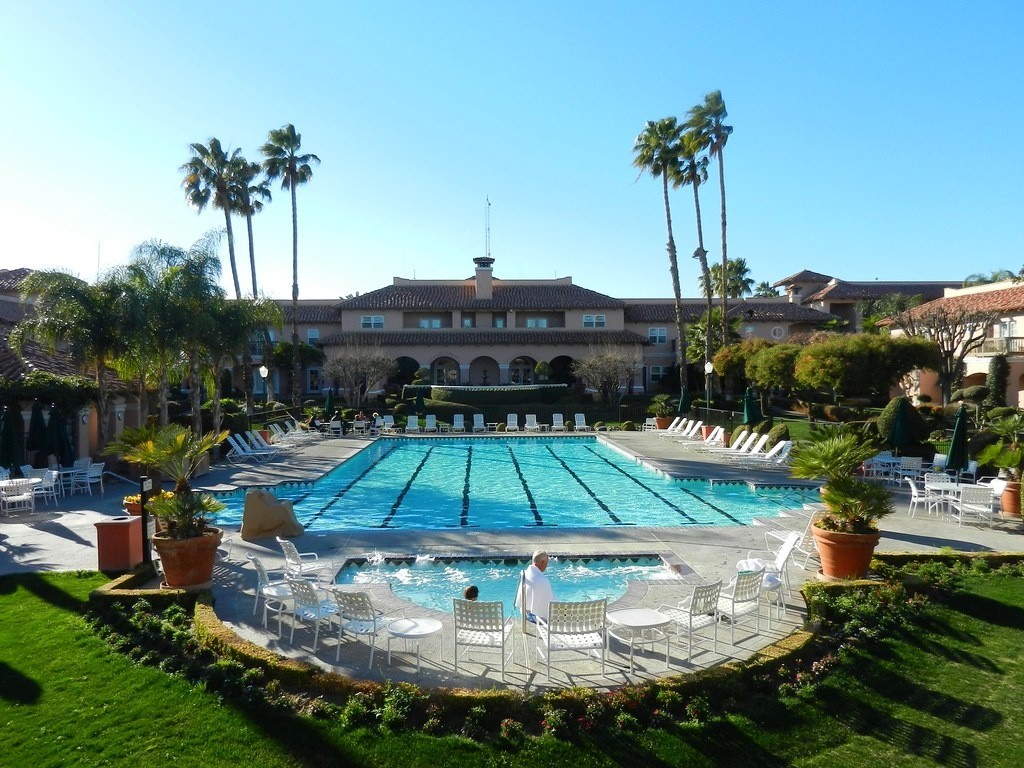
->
[124,489,172,501]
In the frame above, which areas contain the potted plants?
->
[648,393,675,429]
[789,422,895,582]
[100,418,227,587]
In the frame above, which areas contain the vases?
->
[120,501,146,517]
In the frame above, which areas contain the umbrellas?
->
[744,388,756,425]
[325,388,334,416]
[678,387,690,419]
[946,404,968,515]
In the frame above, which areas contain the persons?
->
[464,585,479,601]
[354,411,381,435]
[309,416,319,430]
[331,411,346,435]
[515,550,552,624]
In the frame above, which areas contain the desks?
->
[607,608,672,674]
[1,478,47,511]
[928,482,981,521]
[49,466,87,498]
[488,422,498,433]
[387,616,443,673]
[263,581,320,638]
[875,456,909,485]
[439,424,450,434]
[317,421,372,433]
[539,424,549,432]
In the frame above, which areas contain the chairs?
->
[473,414,486,433]
[0,459,105,516]
[404,416,420,434]
[226,415,401,461]
[505,414,519,434]
[534,598,608,681]
[425,415,438,434]
[525,414,539,433]
[642,418,792,471]
[453,414,465,434]
[246,536,406,670]
[551,414,569,433]
[453,597,516,681]
[574,414,592,432]
[863,450,1009,527]
[659,508,829,664]
